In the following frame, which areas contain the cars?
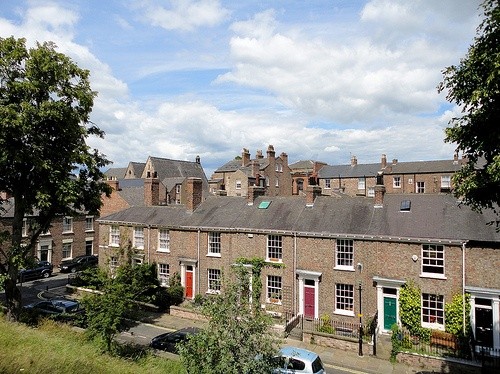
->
[149,327,214,357]
[56,254,98,274]
[252,346,326,374]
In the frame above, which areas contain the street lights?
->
[355,277,364,357]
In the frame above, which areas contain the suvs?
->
[26,299,81,323]
[15,260,53,283]
